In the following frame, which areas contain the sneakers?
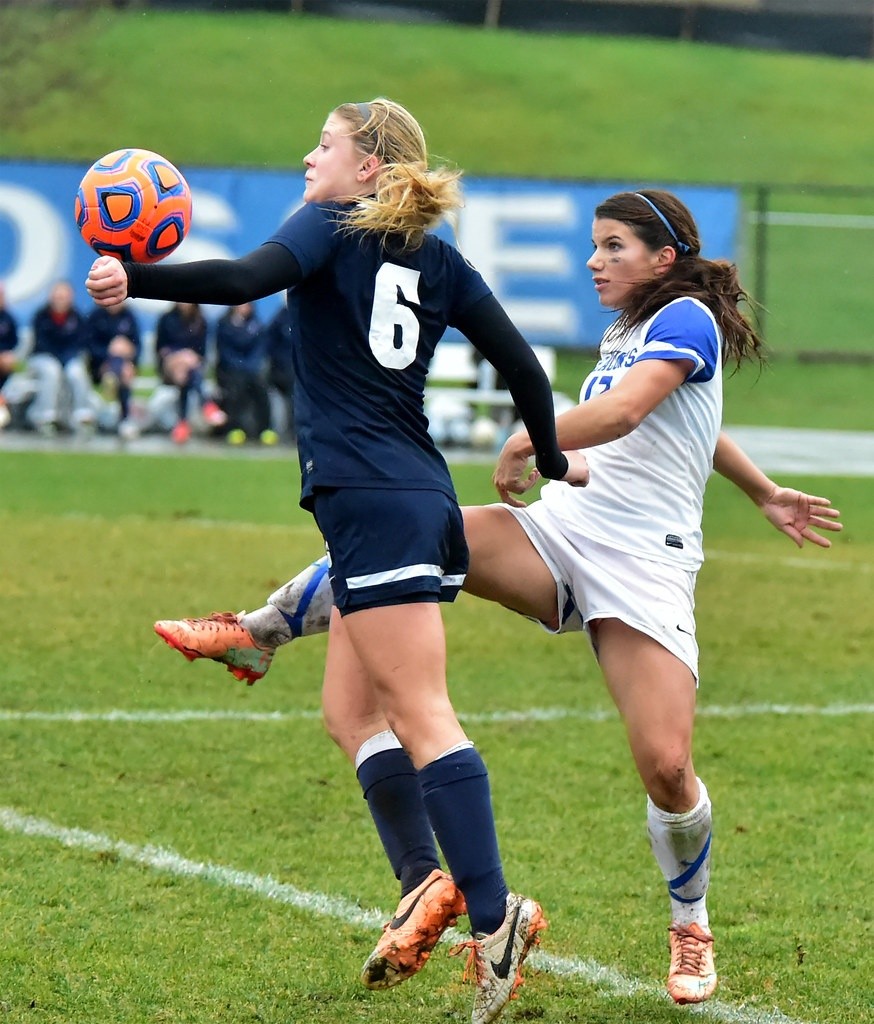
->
[362,869,468,990]
[448,892,547,1024]
[152,610,276,685]
[667,920,717,1004]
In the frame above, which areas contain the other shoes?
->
[0,402,281,445]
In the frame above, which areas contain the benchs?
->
[131,334,559,429]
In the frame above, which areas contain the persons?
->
[155,190,842,1005]
[157,300,297,447]
[85,97,591,1024]
[0,281,142,439]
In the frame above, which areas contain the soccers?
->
[74,149,192,265]
